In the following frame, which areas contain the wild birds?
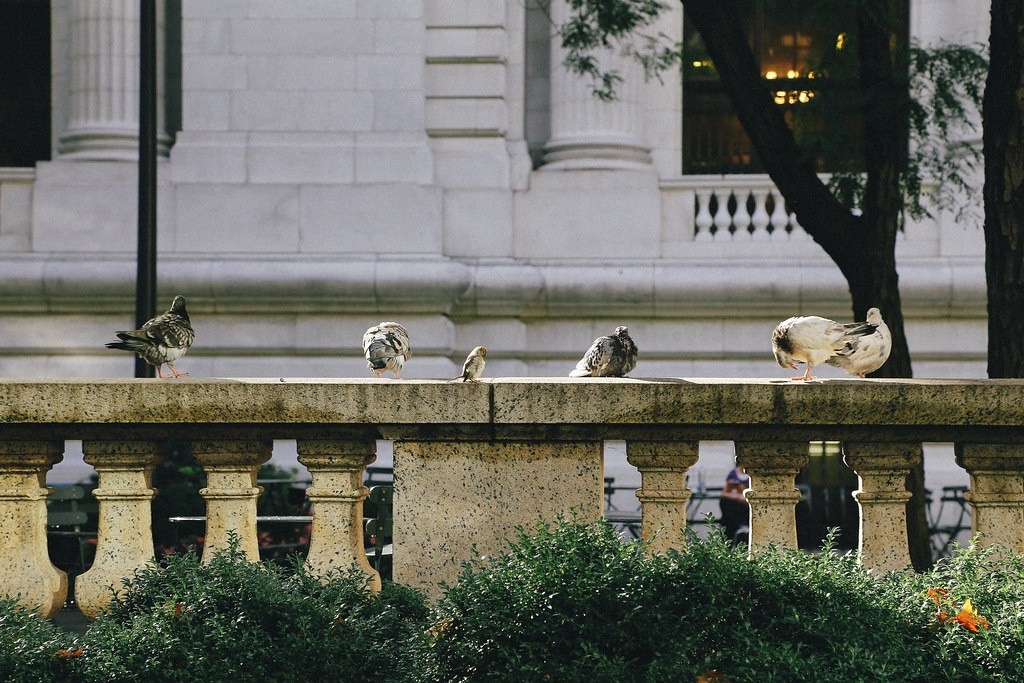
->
[362,322,412,378]
[105,295,194,379]
[449,346,488,382]
[770,308,892,382]
[568,325,638,377]
